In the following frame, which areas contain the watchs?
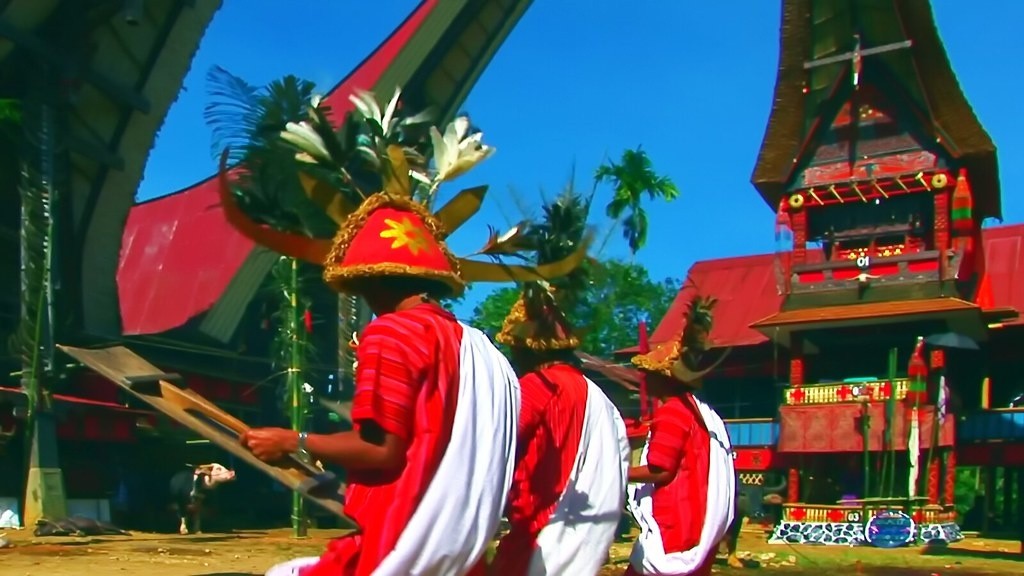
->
[296,431,312,464]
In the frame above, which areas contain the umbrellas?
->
[923,331,980,350]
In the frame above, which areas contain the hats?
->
[493,278,581,353]
[630,292,733,392]
[216,86,593,294]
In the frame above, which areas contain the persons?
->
[239,193,521,576]
[488,285,631,576]
[623,333,735,576]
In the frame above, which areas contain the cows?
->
[33,518,133,537]
[169,462,237,535]
